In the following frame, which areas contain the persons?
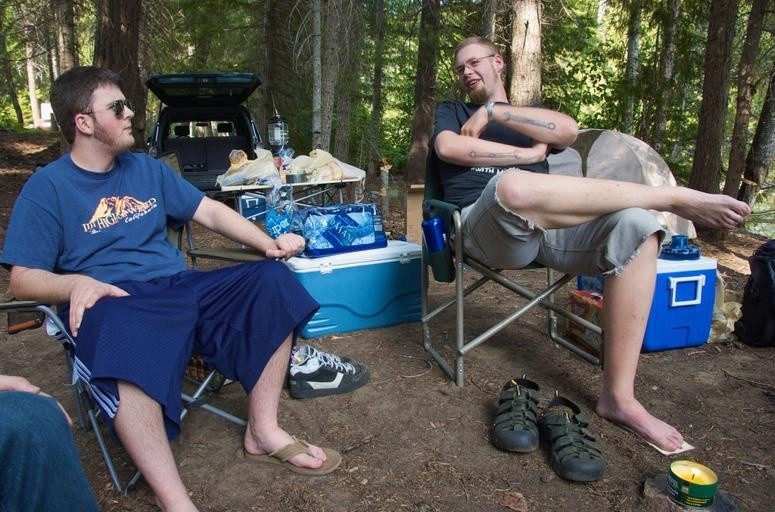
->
[0,373,101,511]
[428,35,754,452]
[0,64,330,511]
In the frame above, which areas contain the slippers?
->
[243,435,342,476]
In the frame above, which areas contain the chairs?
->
[170,124,241,168]
[0,218,281,497]
[420,201,603,388]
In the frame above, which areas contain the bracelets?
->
[486,101,494,122]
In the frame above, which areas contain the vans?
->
[145,74,267,201]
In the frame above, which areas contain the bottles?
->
[291,210,308,255]
[314,209,371,249]
[420,209,446,254]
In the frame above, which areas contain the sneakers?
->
[293,344,351,363]
[289,356,366,397]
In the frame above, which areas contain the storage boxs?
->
[283,240,429,339]
[576,246,718,353]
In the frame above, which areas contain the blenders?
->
[265,111,293,175]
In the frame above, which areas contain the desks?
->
[220,178,362,219]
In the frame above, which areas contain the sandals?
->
[491,377,538,453]
[539,398,604,482]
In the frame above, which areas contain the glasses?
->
[81,100,136,120]
[455,54,496,75]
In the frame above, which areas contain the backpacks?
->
[734,240,775,349]
